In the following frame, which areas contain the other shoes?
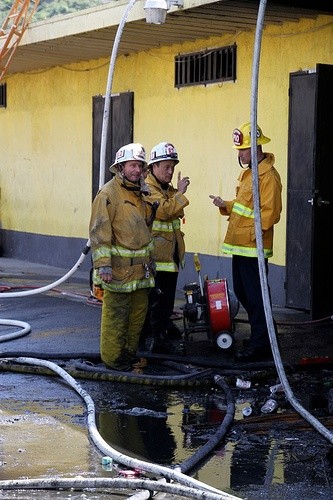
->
[121,367,143,374]
[128,358,147,368]
[234,350,254,361]
[143,339,179,355]
[243,337,250,345]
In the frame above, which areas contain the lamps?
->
[143,0,184,25]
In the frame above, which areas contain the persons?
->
[209,122,282,363]
[89,143,157,375]
[140,142,189,357]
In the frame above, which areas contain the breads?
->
[269,383,285,393]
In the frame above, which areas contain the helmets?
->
[233,123,271,149]
[109,143,147,173]
[147,143,179,168]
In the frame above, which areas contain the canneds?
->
[242,406,253,417]
[261,400,278,413]
[236,378,251,388]
